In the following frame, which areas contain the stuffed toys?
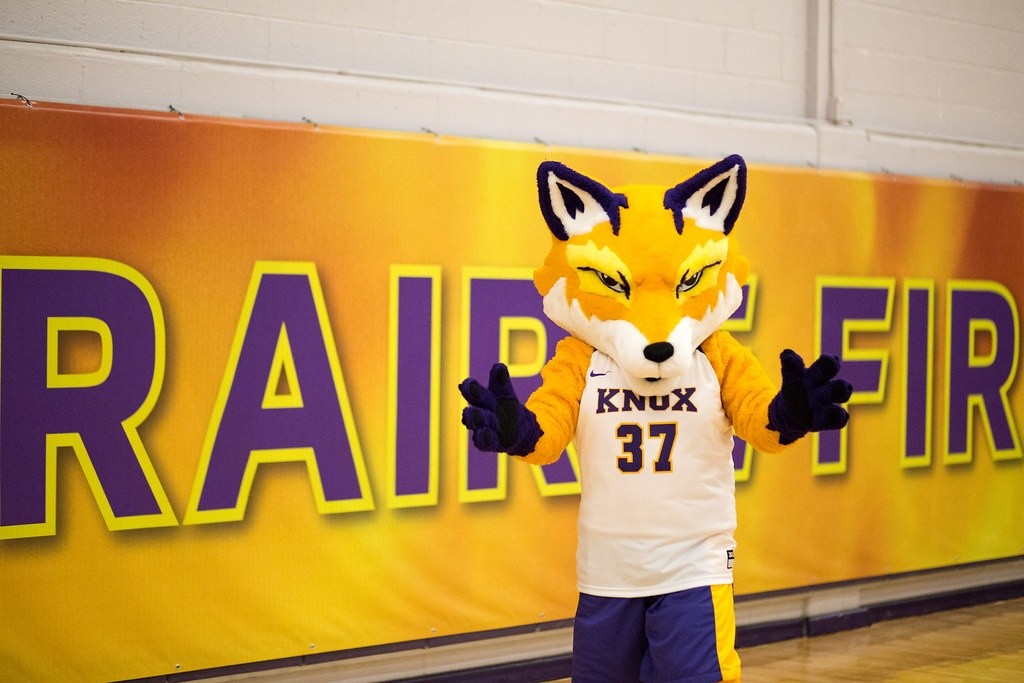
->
[458,155,853,683]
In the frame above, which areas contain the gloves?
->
[767,350,854,447]
[458,362,545,457]
